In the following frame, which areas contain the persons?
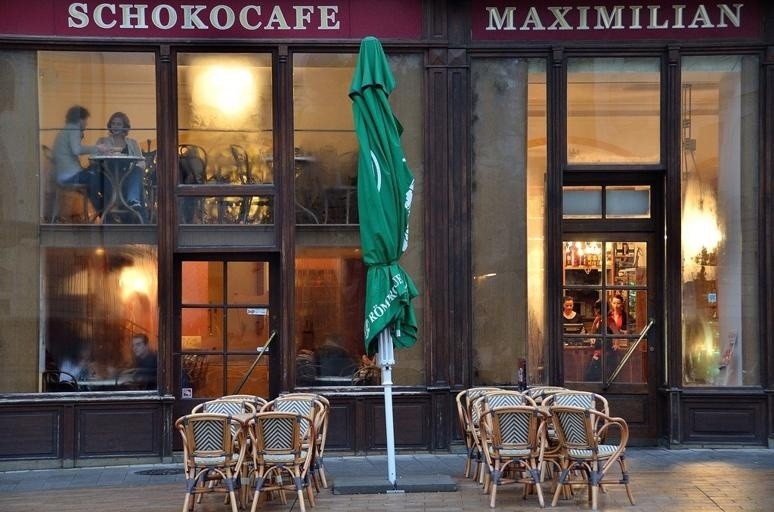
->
[127,335,158,390]
[52,106,113,224]
[607,294,635,334]
[563,296,586,335]
[586,300,621,380]
[92,114,146,225]
[59,339,97,392]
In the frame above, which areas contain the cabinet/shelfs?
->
[265,258,343,351]
[562,242,617,321]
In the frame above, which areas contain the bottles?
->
[564,238,602,267]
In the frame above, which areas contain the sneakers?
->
[128,200,143,213]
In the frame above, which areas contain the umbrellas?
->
[347,37,419,487]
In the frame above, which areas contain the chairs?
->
[295,345,382,386]
[175,393,332,512]
[456,386,637,511]
[42,354,209,392]
